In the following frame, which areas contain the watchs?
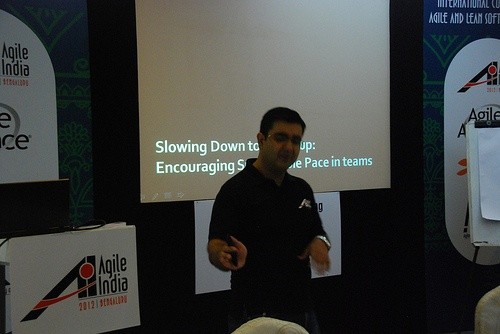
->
[316,234,330,250]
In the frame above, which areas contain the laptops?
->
[0,179,70,239]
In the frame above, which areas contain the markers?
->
[226,233,238,268]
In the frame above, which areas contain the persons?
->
[207,106,331,334]
